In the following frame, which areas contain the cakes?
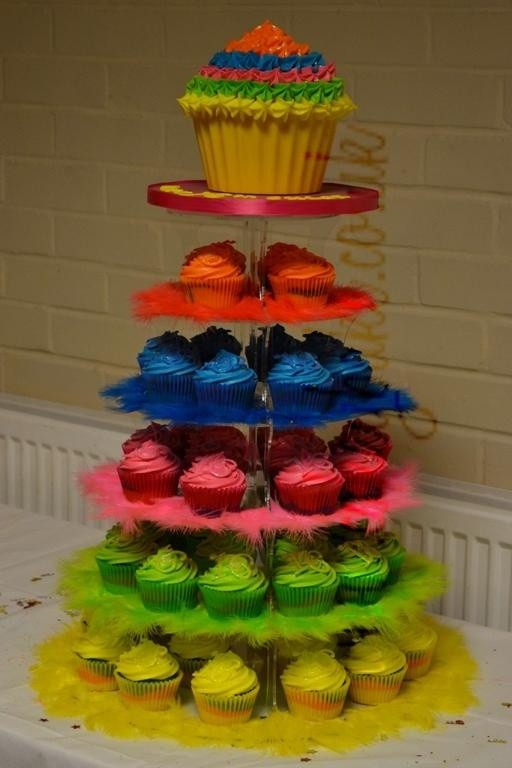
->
[70,323,440,730]
[176,20,353,197]
[258,243,335,309]
[180,239,247,309]
